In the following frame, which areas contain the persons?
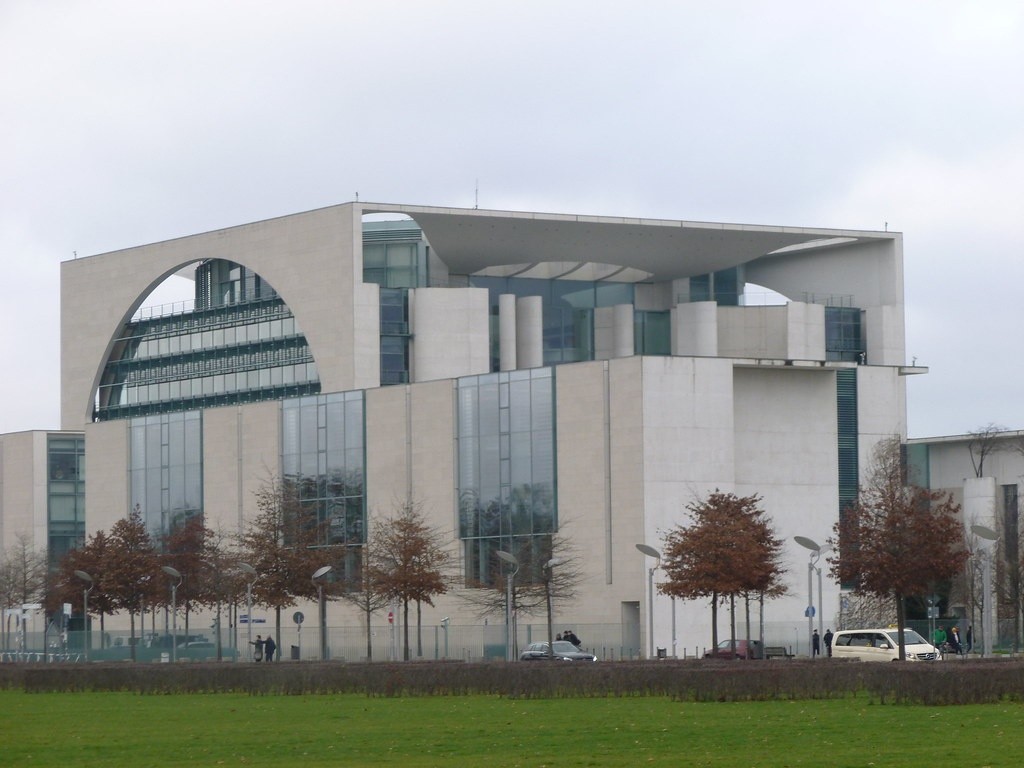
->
[563,630,577,645]
[812,629,819,657]
[966,626,976,653]
[951,625,963,655]
[249,635,263,662]
[556,633,562,641]
[932,625,947,649]
[106,632,110,648]
[258,633,276,662]
[824,629,834,658]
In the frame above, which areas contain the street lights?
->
[807,543,832,658]
[312,564,333,661]
[238,562,258,661]
[635,543,662,660]
[496,550,521,663]
[541,556,572,661]
[793,535,822,660]
[74,568,96,663]
[161,565,182,662]
[969,524,1001,658]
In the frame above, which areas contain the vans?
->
[829,626,944,663]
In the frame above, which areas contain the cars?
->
[519,641,598,663]
[176,641,216,659]
[701,638,756,661]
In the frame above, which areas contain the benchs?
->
[765,647,795,660]
